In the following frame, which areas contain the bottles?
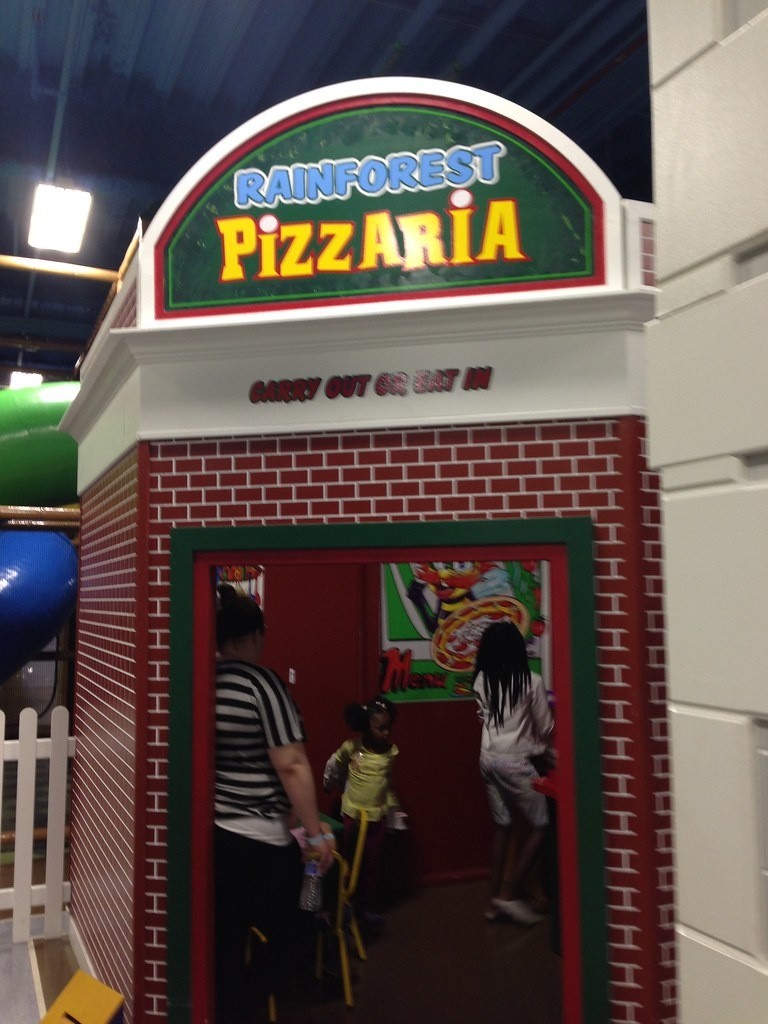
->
[299,849,322,911]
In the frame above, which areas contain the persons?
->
[473,621,554,926]
[213,584,330,1024]
[323,695,400,925]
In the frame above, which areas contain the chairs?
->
[246,808,368,1022]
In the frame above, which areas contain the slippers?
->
[482,898,542,928]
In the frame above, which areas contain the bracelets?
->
[306,833,323,846]
